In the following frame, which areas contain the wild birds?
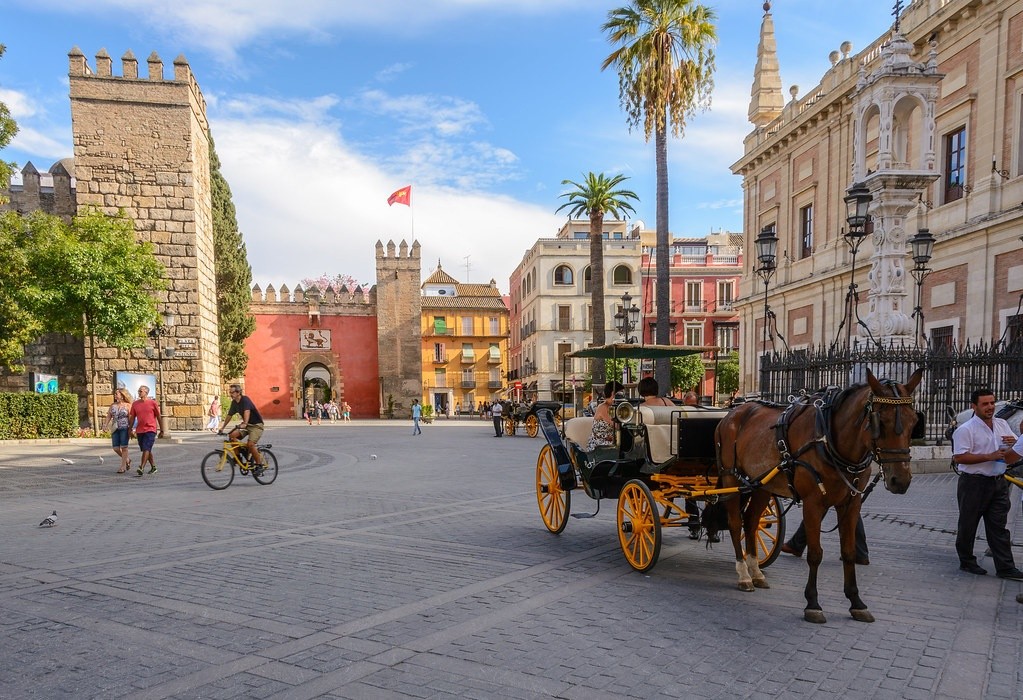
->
[61,456,105,465]
[370,454,378,460]
[39,509,58,527]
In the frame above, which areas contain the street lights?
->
[753,223,789,355]
[911,228,937,351]
[614,292,640,399]
[830,182,880,349]
[146,305,178,439]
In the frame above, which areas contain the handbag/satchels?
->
[207,409,215,418]
[111,421,118,434]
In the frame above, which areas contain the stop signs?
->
[514,381,522,389]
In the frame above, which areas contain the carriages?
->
[530,344,927,623]
[488,399,563,438]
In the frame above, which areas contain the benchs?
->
[635,406,728,466]
[564,416,595,451]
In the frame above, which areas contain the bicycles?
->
[201,425,279,490]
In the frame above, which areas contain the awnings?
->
[495,381,536,396]
[434,317,447,334]
[461,343,500,359]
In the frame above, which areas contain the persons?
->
[206,396,220,432]
[468,401,474,419]
[478,400,518,421]
[411,399,422,436]
[637,376,677,407]
[492,398,502,437]
[219,385,266,476]
[445,401,449,419]
[436,401,443,418]
[102,385,164,474]
[302,400,352,425]
[952,389,1023,604]
[454,401,461,420]
[585,381,624,452]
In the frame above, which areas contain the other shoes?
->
[960,565,987,575]
[998,570,1023,582]
[840,556,869,565]
[1016,593,1023,604]
[413,432,416,436]
[137,465,144,475]
[494,435,502,437]
[781,543,802,557]
[227,456,242,464]
[418,432,421,435]
[711,533,720,542]
[148,465,158,474]
[253,464,263,476]
[117,468,126,473]
[126,459,131,470]
[689,530,699,540]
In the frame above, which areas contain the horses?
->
[700,368,924,623]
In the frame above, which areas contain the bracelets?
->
[160,430,164,433]
[242,422,248,425]
[103,424,107,427]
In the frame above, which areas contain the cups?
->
[999,445,1009,451]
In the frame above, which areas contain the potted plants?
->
[421,405,435,424]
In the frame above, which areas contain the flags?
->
[387,185,411,207]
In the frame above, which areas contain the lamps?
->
[782,246,795,262]
[953,171,972,193]
[841,225,855,240]
[752,261,756,272]
[991,154,1011,180]
[525,357,537,371]
[918,193,933,209]
[500,369,509,378]
[804,237,815,253]
